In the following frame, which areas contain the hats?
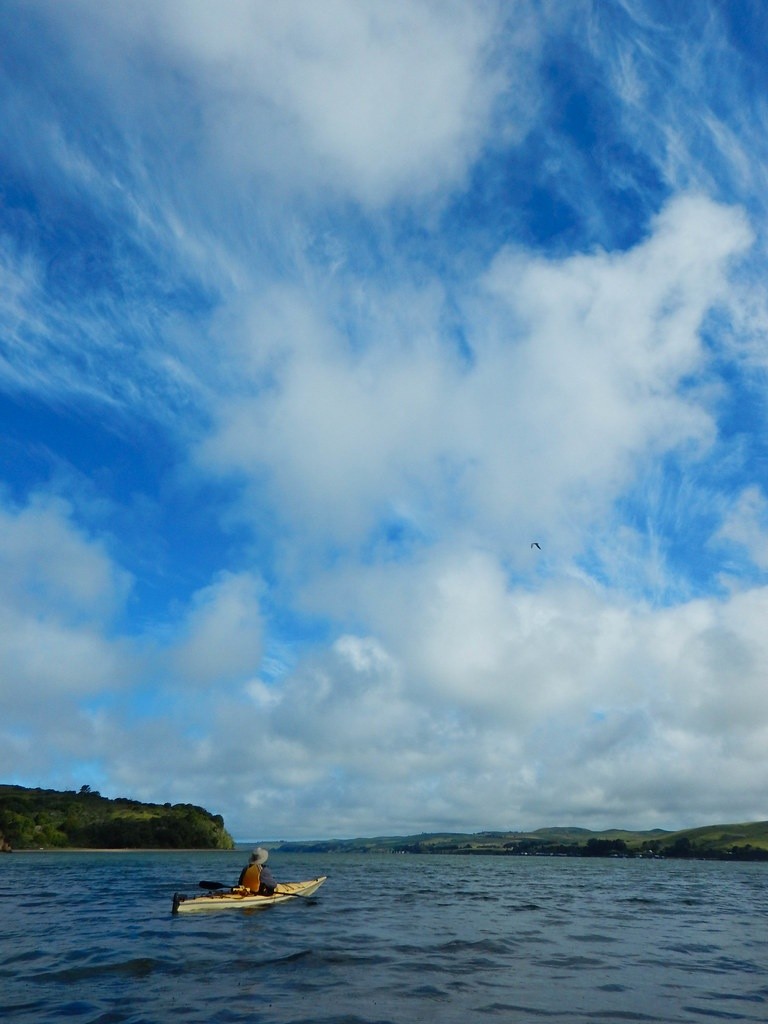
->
[248,848,268,864]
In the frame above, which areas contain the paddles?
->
[198,880,323,901]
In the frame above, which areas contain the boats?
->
[170,876,327,915]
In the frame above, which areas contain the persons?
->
[238,847,278,897]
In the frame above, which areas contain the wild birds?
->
[531,543,541,550]
